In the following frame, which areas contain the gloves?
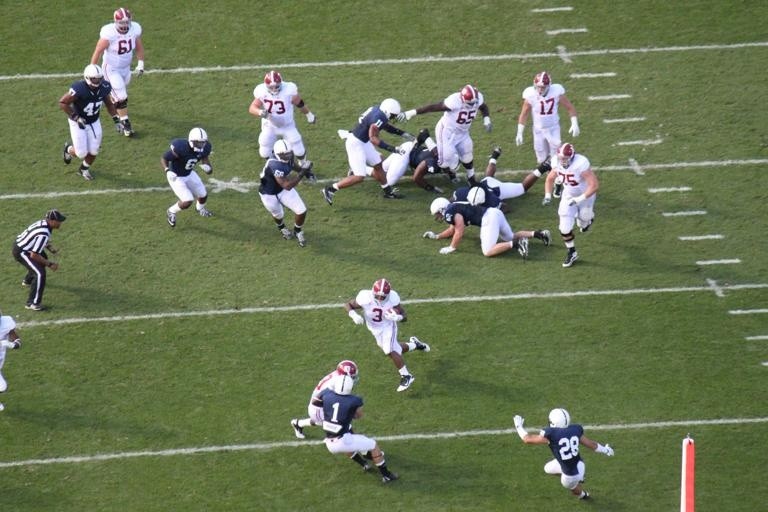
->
[440,247,456,254]
[307,174,316,184]
[394,146,406,156]
[541,193,551,208]
[259,109,269,119]
[199,164,210,172]
[306,111,317,125]
[303,160,313,171]
[70,112,87,129]
[112,115,124,135]
[402,132,415,141]
[569,194,586,206]
[349,310,364,325]
[1,339,16,348]
[513,414,528,440]
[397,109,417,123]
[515,123,525,147]
[385,309,403,322]
[134,60,144,78]
[423,231,438,240]
[167,171,178,182]
[483,116,493,133]
[426,184,443,193]
[568,116,580,138]
[596,443,614,457]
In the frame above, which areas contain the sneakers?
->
[291,418,305,438]
[542,229,551,246]
[166,209,175,226]
[539,155,552,167]
[25,303,44,311]
[320,186,334,206]
[76,167,94,180]
[293,227,305,248]
[277,224,291,239]
[364,465,375,474]
[382,472,398,482]
[495,146,502,153]
[397,375,415,392]
[517,237,528,257]
[579,490,589,500]
[465,173,475,186]
[580,215,593,232]
[409,336,430,353]
[418,128,429,145]
[384,191,405,199]
[22,279,32,286]
[64,142,71,163]
[121,119,134,136]
[562,251,580,267]
[195,206,212,217]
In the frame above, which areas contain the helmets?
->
[430,197,451,223]
[114,8,132,32]
[264,71,282,95]
[274,139,293,162]
[449,152,460,171]
[335,375,353,395]
[84,64,104,87]
[189,128,208,152]
[557,142,576,168]
[372,278,390,305]
[548,408,570,427]
[533,72,551,96]
[379,98,401,120]
[467,187,485,206]
[461,85,478,108]
[337,360,357,383]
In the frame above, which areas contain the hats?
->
[46,208,66,221]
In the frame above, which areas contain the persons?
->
[312,375,400,484]
[0,311,21,412]
[345,277,430,393]
[514,408,615,499]
[320,84,552,260]
[250,71,317,184]
[58,64,123,181]
[542,142,601,269]
[516,72,580,197]
[291,360,358,439]
[91,7,145,137]
[259,139,316,248]
[160,127,214,228]
[12,209,66,310]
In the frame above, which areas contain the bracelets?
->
[48,262,52,267]
